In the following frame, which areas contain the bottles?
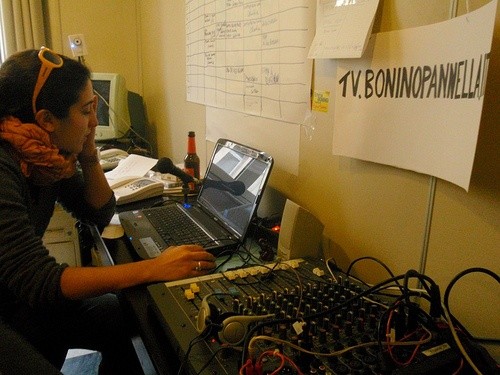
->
[183,131,200,195]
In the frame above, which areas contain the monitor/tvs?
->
[87,73,131,142]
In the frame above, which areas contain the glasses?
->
[33,46,63,114]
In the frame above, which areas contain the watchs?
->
[78,149,101,165]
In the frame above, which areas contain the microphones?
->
[157,157,199,184]
[204,180,246,196]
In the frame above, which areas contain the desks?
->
[77,220,188,375]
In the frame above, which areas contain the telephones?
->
[96,148,130,170]
[109,175,165,206]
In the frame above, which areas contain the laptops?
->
[119,138,274,263]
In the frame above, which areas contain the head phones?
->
[196,293,275,346]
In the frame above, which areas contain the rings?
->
[195,262,202,272]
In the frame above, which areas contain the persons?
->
[0,46,216,375]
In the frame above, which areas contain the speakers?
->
[276,198,324,261]
